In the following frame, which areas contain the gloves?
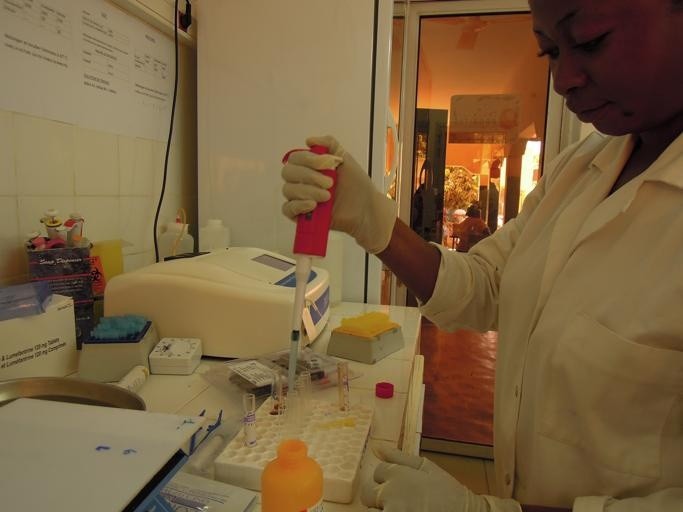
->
[282,136,398,254]
[360,446,523,512]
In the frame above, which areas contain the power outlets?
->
[178,10,188,33]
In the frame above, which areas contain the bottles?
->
[157,219,194,263]
[270,368,313,445]
[336,361,350,411]
[242,391,258,448]
[198,217,230,255]
[372,380,397,438]
[257,440,327,512]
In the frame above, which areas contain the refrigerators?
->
[193,0,413,308]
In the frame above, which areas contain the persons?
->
[281,2,682,511]
[453,205,492,253]
